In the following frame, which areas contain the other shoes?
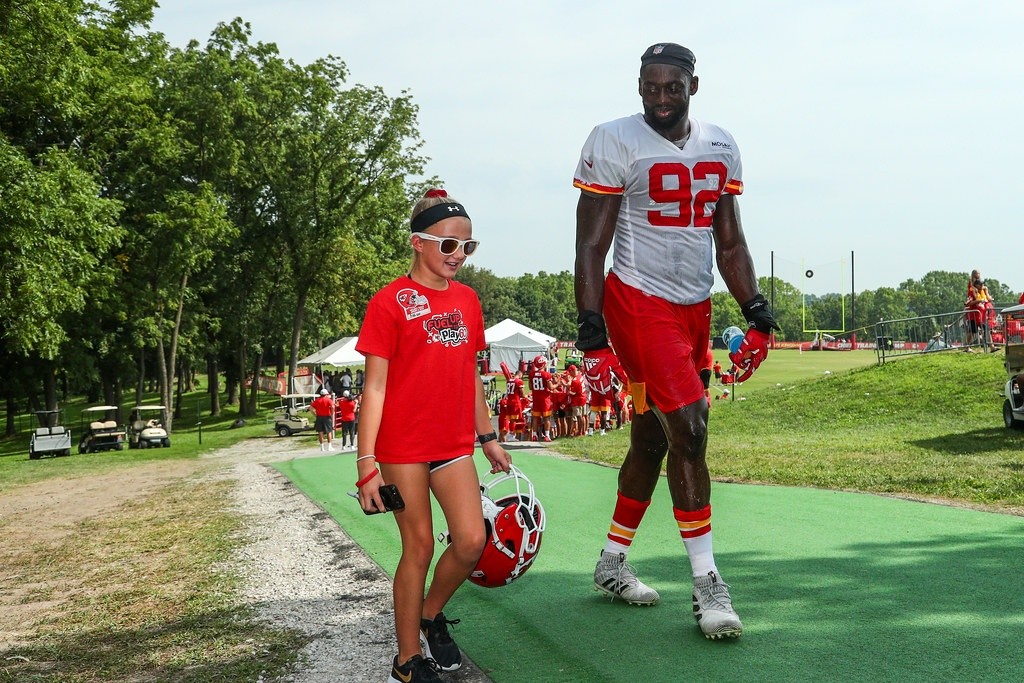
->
[342,446,345,450]
[321,448,326,452]
[498,420,624,442]
[328,447,335,451]
[990,347,1001,353]
[351,446,355,450]
[965,348,973,352]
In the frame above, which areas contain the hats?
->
[343,390,350,397]
[320,389,329,395]
[974,279,985,285]
[567,365,578,373]
[640,42,696,77]
[932,332,943,338]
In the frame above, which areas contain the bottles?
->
[722,325,751,370]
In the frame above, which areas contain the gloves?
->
[728,294,780,382]
[573,309,629,404]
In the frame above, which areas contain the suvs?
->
[565,354,583,369]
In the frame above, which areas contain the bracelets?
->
[356,455,377,462]
[478,430,497,444]
[355,468,379,488]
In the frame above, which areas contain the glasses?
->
[410,232,480,257]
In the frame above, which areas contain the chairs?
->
[36,426,65,436]
[287,407,298,417]
[90,421,118,430]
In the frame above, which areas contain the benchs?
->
[133,419,162,429]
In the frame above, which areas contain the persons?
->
[573,41,784,639]
[963,269,1002,353]
[700,342,741,408]
[311,368,365,450]
[498,355,635,442]
[356,189,514,683]
[924,332,955,350]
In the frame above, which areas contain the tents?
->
[484,318,557,373]
[297,337,366,389]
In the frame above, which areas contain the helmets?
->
[534,356,547,369]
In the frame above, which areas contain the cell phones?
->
[356,484,405,515]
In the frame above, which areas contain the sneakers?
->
[593,552,660,606]
[691,570,744,639]
[387,655,444,683]
[419,598,464,671]
